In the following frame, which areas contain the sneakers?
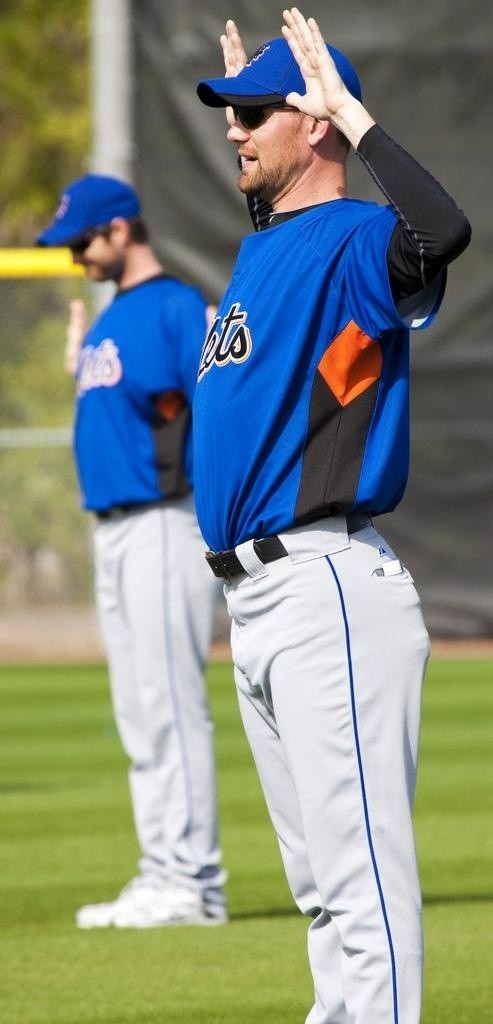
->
[73,888,228,929]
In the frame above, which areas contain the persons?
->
[35,173,229,927]
[187,7,471,1024]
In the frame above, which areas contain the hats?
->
[32,174,142,247]
[193,35,362,108]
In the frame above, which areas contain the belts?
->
[204,513,375,579]
[95,496,190,528]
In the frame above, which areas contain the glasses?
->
[231,102,295,128]
